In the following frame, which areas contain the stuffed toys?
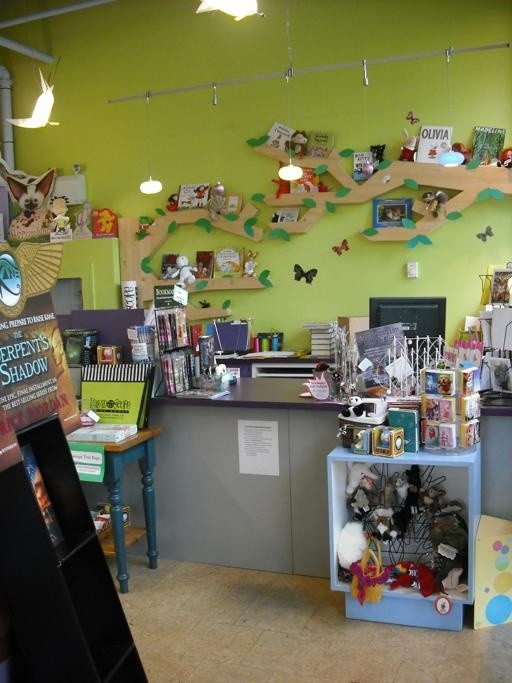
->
[341,395,368,418]
[399,129,419,162]
[423,190,449,217]
[208,181,226,220]
[354,430,366,449]
[286,130,308,158]
[170,255,198,289]
[501,148,512,168]
[337,462,468,597]
[380,429,391,448]
[453,143,472,165]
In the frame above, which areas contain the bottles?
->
[254,334,278,352]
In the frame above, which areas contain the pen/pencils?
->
[130,326,153,344]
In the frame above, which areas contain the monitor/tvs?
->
[370,297,445,371]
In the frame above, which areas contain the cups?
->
[121,280,138,308]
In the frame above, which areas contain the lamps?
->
[107,43,511,195]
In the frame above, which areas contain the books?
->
[188,323,215,381]
[266,122,296,151]
[178,183,210,208]
[175,389,230,400]
[472,125,507,166]
[388,365,481,453]
[417,125,452,163]
[353,152,372,182]
[303,323,338,359]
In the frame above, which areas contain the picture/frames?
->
[372,200,412,227]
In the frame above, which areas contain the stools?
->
[65,427,164,593]
